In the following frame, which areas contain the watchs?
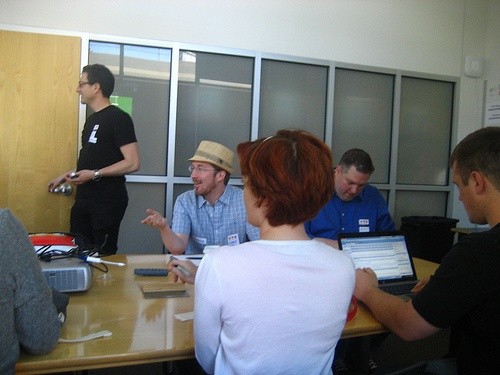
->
[93,169,100,182]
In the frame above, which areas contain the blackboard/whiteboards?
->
[481,79,500,129]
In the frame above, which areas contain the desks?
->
[451,228,493,245]
[14,254,442,375]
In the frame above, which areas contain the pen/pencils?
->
[170,255,191,277]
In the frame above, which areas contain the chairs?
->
[28,233,79,257]
[399,214,459,263]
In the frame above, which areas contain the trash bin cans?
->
[399,216,459,265]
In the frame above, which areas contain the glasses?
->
[79,80,102,89]
[188,166,221,173]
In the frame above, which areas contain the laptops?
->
[337,231,419,304]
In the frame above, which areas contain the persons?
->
[48,63,141,256]
[0,208,62,375]
[141,140,261,255]
[305,147,396,250]
[168,128,356,375]
[355,127,500,375]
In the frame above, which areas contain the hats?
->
[188,141,234,175]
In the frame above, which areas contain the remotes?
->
[134,268,168,276]
